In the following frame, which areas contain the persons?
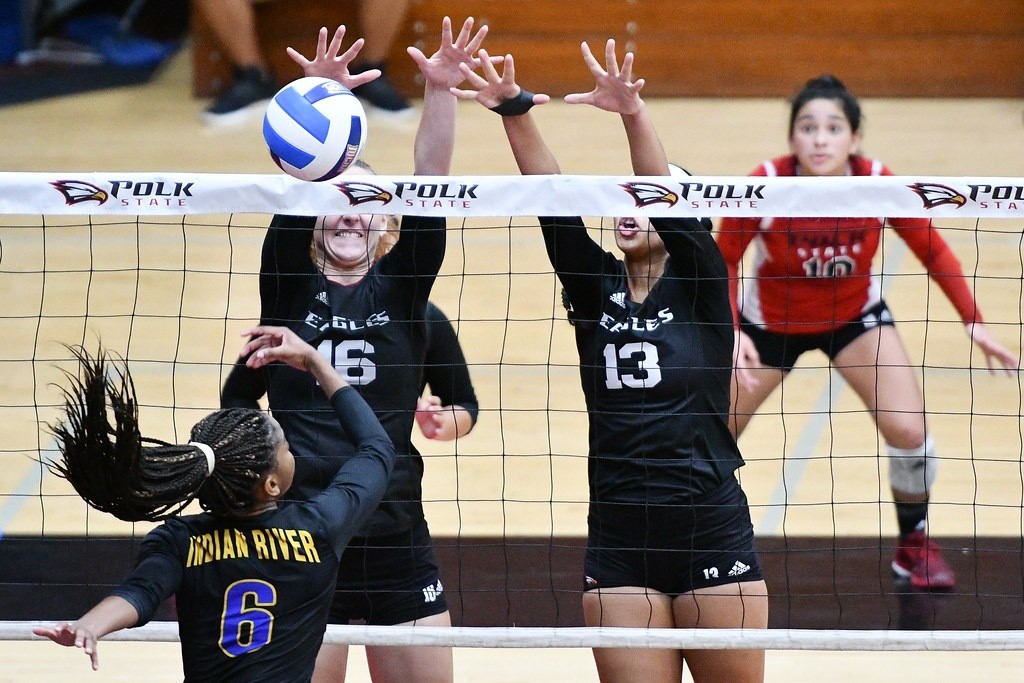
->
[192,0,416,129]
[259,15,504,683]
[220,216,478,441]
[715,76,1018,587]
[449,38,768,683]
[25,325,396,683]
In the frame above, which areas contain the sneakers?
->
[891,531,956,587]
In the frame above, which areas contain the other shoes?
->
[200,65,281,121]
[350,60,415,116]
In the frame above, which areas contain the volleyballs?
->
[258,76,371,182]
